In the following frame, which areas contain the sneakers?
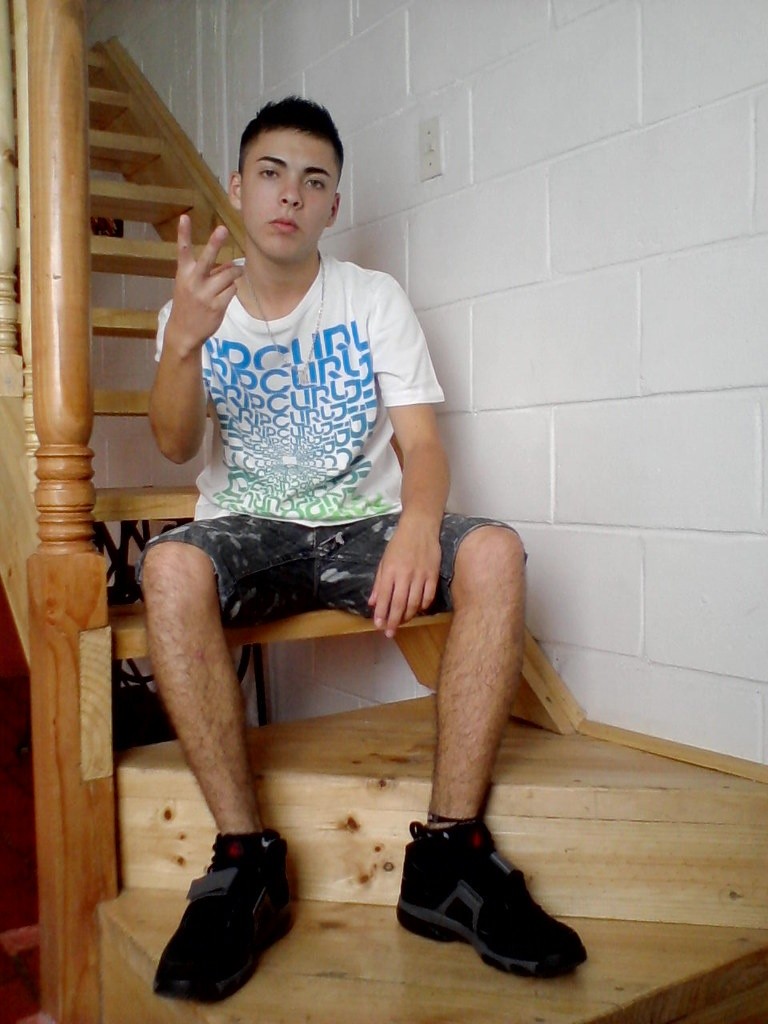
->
[397,815,586,977]
[154,829,291,1002]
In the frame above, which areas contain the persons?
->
[137,94,590,1004]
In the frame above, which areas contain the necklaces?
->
[242,261,327,388]
